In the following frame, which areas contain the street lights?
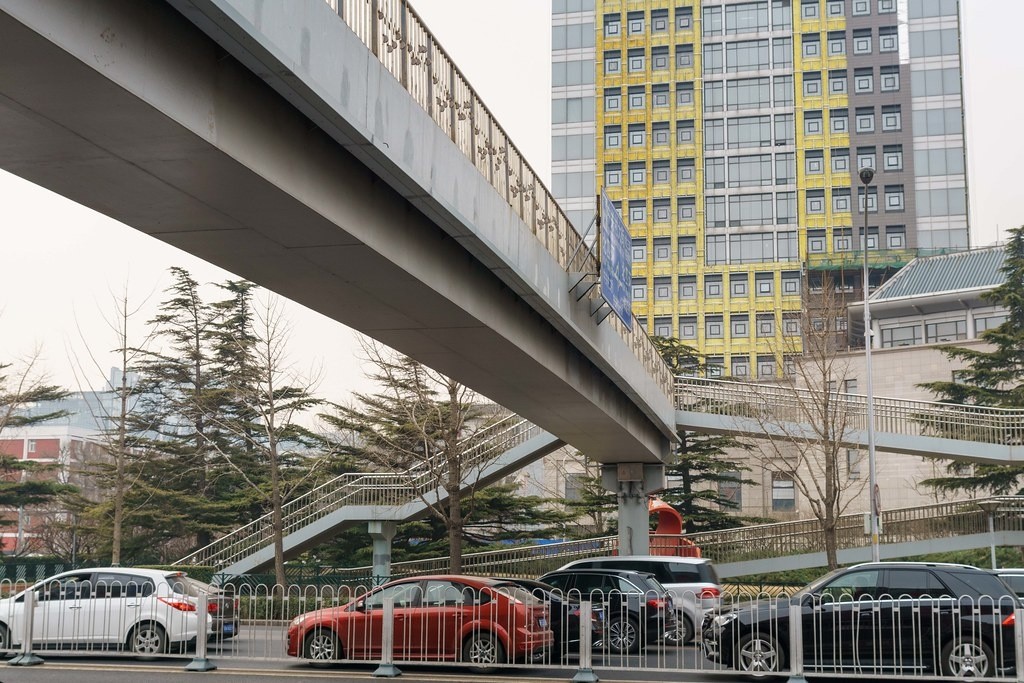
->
[859,166,879,563]
[978,501,1002,569]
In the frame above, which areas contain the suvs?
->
[699,561,1024,683]
[536,568,676,654]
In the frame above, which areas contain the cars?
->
[425,578,609,660]
[285,574,553,675]
[0,568,238,662]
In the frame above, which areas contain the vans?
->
[557,556,722,645]
